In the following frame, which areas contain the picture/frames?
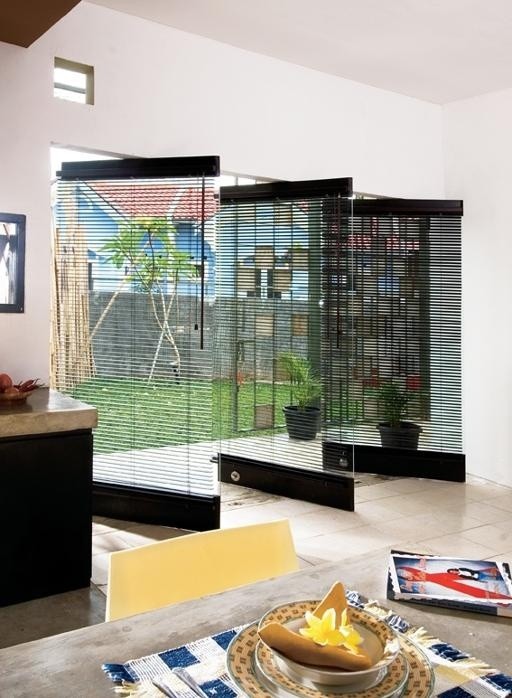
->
[0,212,26,313]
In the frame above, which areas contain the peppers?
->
[13,378,46,393]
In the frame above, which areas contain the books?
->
[385,549,511,618]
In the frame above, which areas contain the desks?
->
[0,387,98,611]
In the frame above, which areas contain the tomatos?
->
[0,373,20,394]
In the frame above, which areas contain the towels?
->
[258,581,373,672]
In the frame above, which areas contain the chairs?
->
[105,517,301,624]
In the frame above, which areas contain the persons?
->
[446,567,502,583]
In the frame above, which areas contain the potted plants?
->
[273,350,325,440]
[371,380,422,451]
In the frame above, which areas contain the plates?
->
[0,389,36,401]
[226,599,436,698]
